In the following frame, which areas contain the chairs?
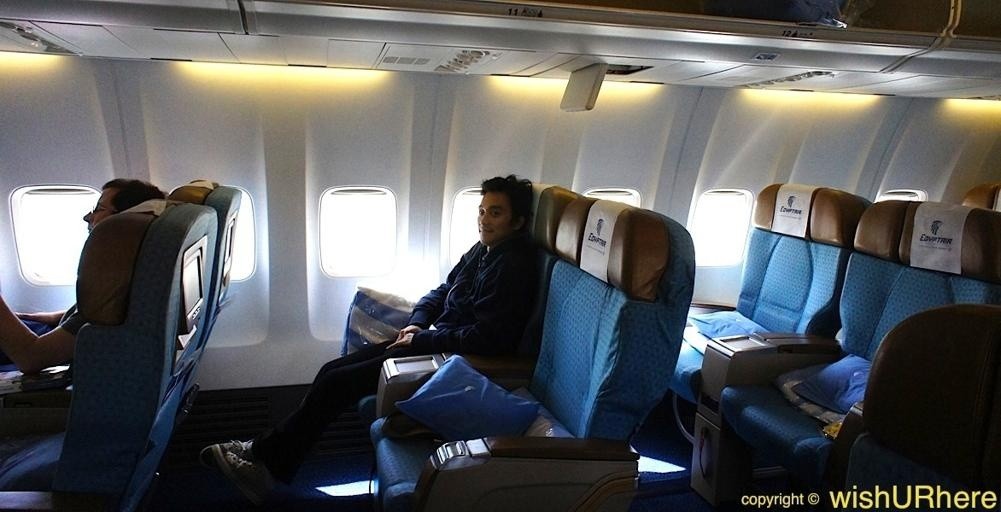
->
[344,183,1001,511]
[0,178,243,511]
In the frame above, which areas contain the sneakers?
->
[212,444,267,507]
[202,439,256,466]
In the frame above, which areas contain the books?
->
[0,367,73,396]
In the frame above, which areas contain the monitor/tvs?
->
[222,216,235,278]
[182,246,205,335]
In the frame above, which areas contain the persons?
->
[0,177,170,372]
[198,175,546,506]
